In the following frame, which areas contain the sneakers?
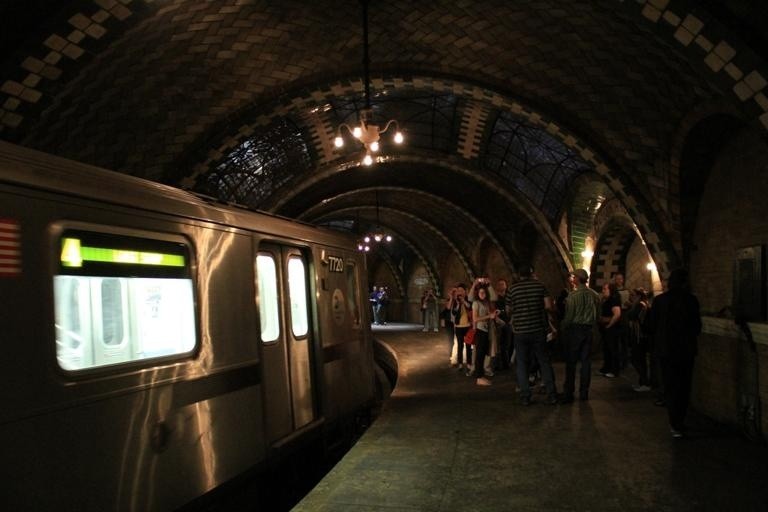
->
[632,383,683,437]
[600,368,616,378]
[373,321,387,325]
[422,328,439,332]
[451,360,510,386]
[516,376,588,406]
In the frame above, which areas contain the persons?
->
[422,288,446,332]
[643,267,701,437]
[446,267,653,407]
[369,286,381,324]
[376,287,387,325]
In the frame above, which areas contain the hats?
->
[664,269,688,282]
[567,269,587,280]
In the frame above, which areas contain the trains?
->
[0,140,377,512]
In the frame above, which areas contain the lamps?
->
[331,0,408,172]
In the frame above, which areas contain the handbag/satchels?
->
[465,328,480,346]
